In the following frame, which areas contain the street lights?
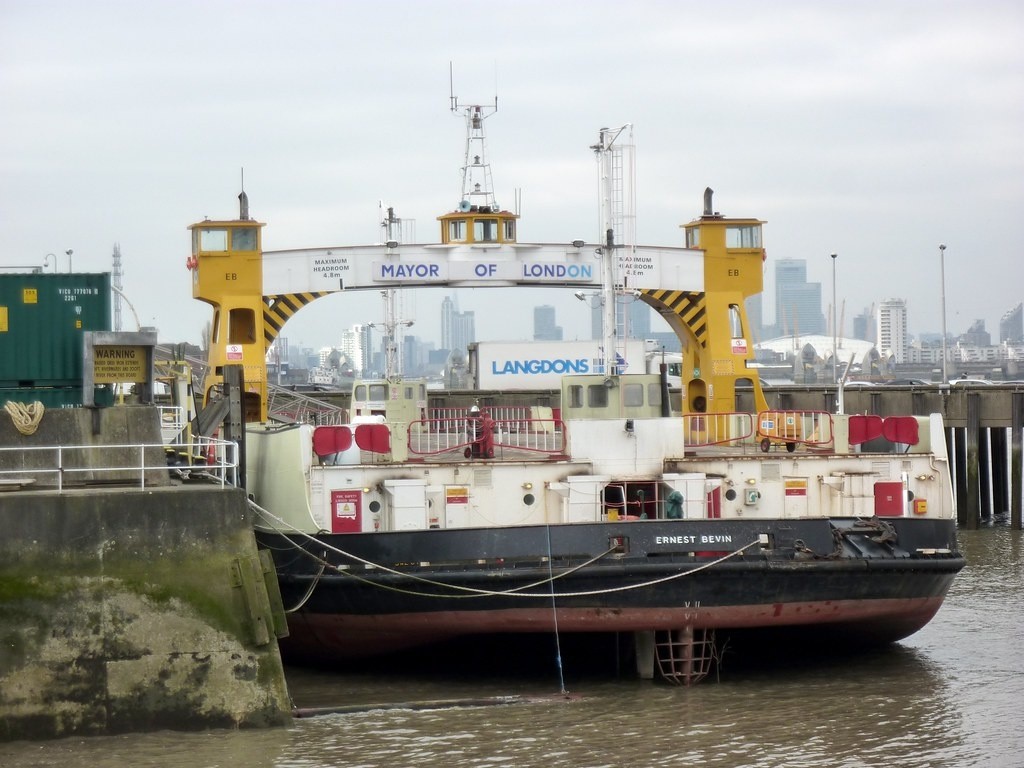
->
[829,251,841,385]
[938,242,951,384]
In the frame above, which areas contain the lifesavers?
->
[208,444,215,464]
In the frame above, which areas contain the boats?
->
[184,54,962,689]
